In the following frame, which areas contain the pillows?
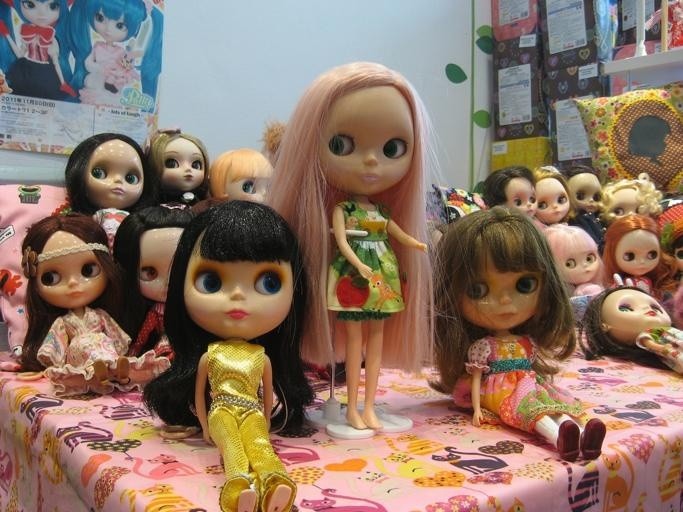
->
[432,182,487,225]
[1,182,70,361]
[568,74,682,202]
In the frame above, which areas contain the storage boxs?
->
[485,0,682,179]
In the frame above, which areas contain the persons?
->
[13,59,683,512]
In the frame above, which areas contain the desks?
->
[1,346,682,512]
